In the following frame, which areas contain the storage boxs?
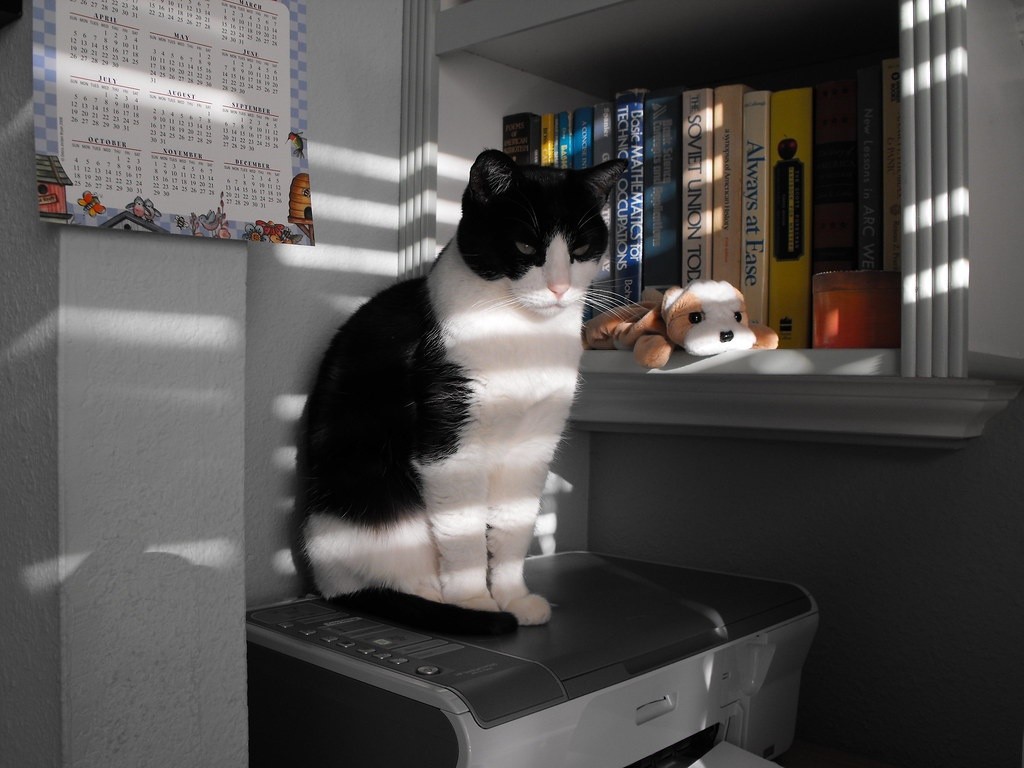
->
[812,270,901,350]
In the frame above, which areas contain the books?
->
[501,57,900,349]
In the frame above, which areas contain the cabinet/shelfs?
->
[401,0,1024,389]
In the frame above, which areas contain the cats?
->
[289,150,632,639]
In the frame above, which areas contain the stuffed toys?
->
[581,278,779,369]
